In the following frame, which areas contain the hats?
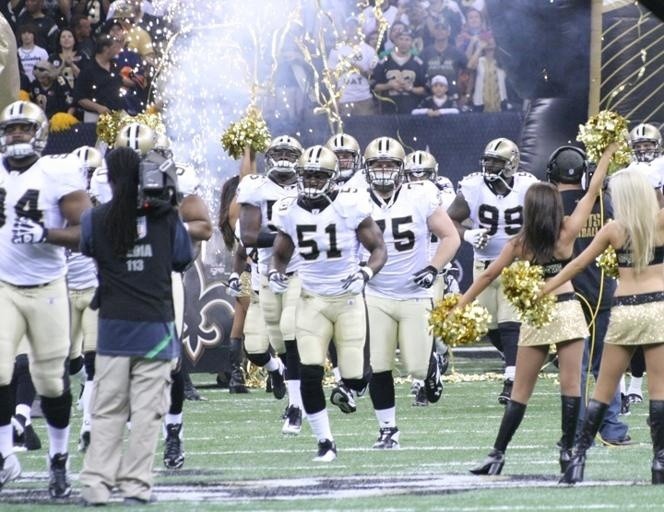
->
[334,0,496,86]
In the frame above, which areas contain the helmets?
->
[0,101,174,172]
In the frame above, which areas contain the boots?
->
[470,395,663,483]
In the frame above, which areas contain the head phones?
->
[547,161,562,178]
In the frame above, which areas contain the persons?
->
[219,132,465,461]
[0,0,496,124]
[530,166,663,487]
[0,100,212,506]
[443,138,542,408]
[542,145,639,448]
[452,140,619,478]
[629,121,664,207]
